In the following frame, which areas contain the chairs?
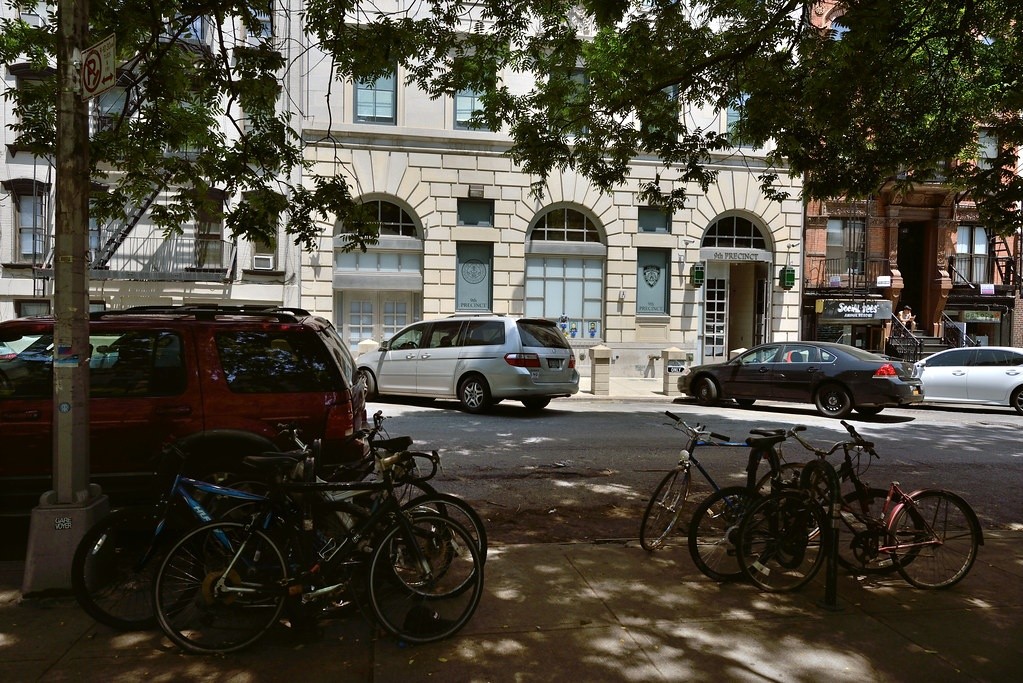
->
[791,352,803,362]
[470,330,484,341]
[440,336,452,347]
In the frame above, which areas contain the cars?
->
[680,341,925,418]
[913,346,1023,415]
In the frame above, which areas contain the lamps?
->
[690,262,705,288]
[780,244,795,290]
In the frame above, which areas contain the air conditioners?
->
[245,34,266,47]
[254,256,273,269]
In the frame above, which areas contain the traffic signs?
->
[667,366,685,374]
[80,34,117,103]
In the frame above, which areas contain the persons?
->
[895,305,916,337]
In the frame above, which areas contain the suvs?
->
[353,312,580,413]
[0,302,375,518]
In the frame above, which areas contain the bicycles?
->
[687,420,925,583]
[638,410,831,554]
[69,407,490,658]
[736,423,986,595]
